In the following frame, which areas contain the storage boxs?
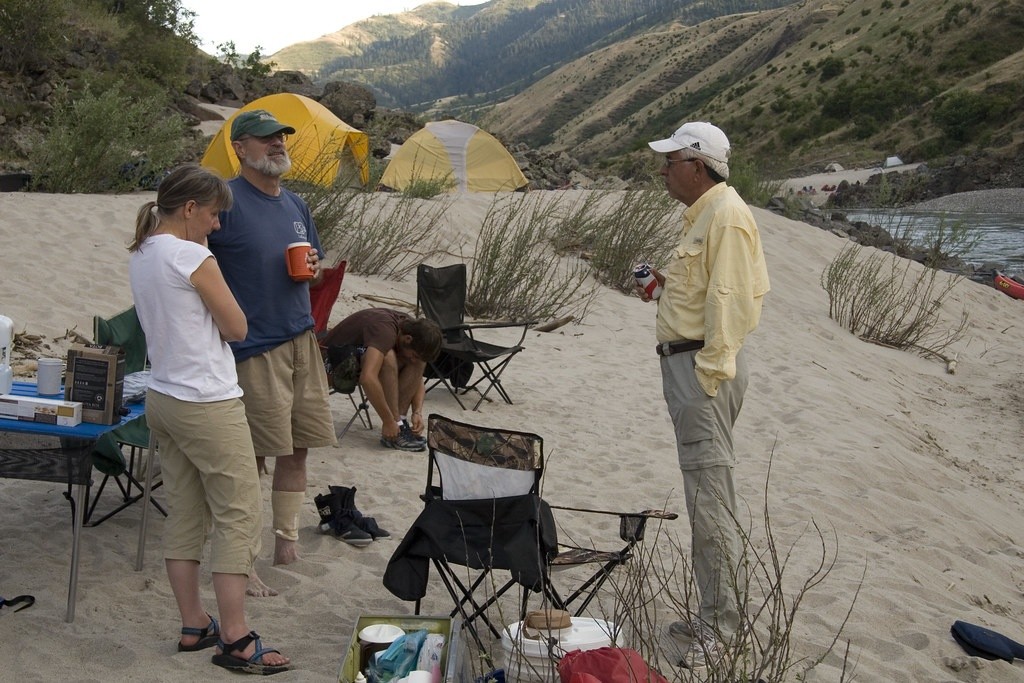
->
[65,342,125,425]
[336,613,471,683]
[0,394,83,427]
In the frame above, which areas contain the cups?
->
[284,242,315,282]
[37,358,66,397]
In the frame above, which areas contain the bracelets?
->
[414,411,422,417]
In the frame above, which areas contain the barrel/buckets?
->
[502,617,624,682]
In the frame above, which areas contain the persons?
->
[319,308,443,451]
[126,165,293,675]
[634,122,771,649]
[201,110,338,599]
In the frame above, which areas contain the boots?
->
[314,485,392,544]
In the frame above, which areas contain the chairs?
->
[417,263,538,411]
[309,259,372,438]
[82,303,167,528]
[415,414,678,672]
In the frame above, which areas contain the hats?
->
[648,122,731,163]
[230,110,295,141]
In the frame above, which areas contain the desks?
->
[0,382,155,623]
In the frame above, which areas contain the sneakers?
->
[380,419,427,452]
[669,619,727,669]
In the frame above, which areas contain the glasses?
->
[237,128,288,143]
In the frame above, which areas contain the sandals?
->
[211,631,288,675]
[178,612,220,651]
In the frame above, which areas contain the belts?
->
[656,339,707,356]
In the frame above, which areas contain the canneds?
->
[632,263,663,300]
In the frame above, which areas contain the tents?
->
[378,119,529,194]
[201,93,372,194]
